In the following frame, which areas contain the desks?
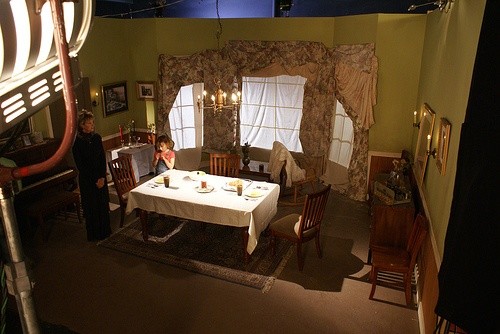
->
[240,158,272,184]
[106,143,157,185]
[127,168,280,267]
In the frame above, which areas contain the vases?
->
[242,151,250,166]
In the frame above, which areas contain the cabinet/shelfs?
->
[367,150,415,265]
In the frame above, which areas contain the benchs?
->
[25,192,82,247]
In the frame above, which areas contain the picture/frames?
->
[22,134,33,145]
[412,103,435,190]
[101,81,157,118]
[437,118,450,176]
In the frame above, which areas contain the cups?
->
[237,185,242,196]
[201,180,206,188]
[164,177,169,187]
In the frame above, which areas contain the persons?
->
[72,109,113,242]
[152,135,175,175]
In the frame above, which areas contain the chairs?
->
[265,184,332,271]
[209,153,241,178]
[264,141,319,205]
[108,156,165,242]
[369,214,428,305]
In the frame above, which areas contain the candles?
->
[96,92,99,106]
[427,134,431,151]
[413,111,417,122]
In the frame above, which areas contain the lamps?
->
[197,0,242,115]
[0,0,94,181]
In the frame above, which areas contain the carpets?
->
[96,214,297,293]
